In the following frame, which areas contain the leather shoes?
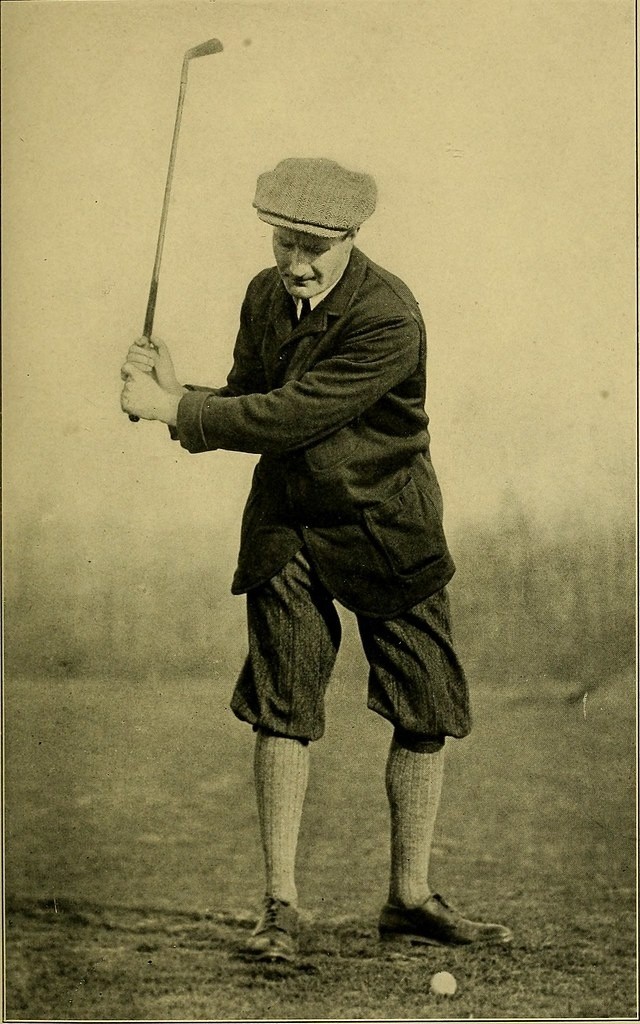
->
[243,895,302,963]
[378,893,512,949]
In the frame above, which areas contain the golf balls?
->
[431,970,457,995]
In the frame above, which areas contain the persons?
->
[118,155,514,962]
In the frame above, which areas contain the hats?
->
[252,157,377,239]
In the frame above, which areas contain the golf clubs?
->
[129,37,226,424]
